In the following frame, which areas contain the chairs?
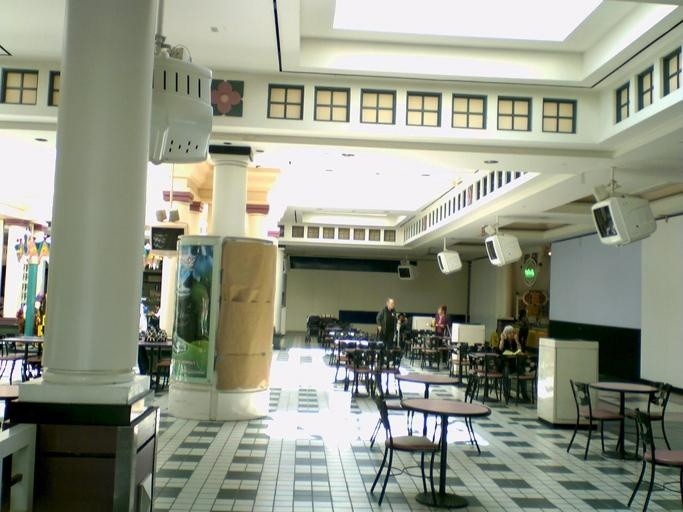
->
[22,355,42,381]
[156,360,171,392]
[0,339,25,384]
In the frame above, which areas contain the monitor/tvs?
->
[485,235,522,266]
[591,196,656,246]
[437,251,462,273]
[150,224,187,255]
[398,266,414,280]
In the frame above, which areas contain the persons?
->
[517,309,528,352]
[431,304,451,364]
[150,300,160,318]
[401,315,410,331]
[375,297,396,365]
[395,312,405,348]
[496,325,532,404]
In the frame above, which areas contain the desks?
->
[145,310,155,325]
[0,385,19,420]
[1,336,44,382]
[139,337,172,392]
